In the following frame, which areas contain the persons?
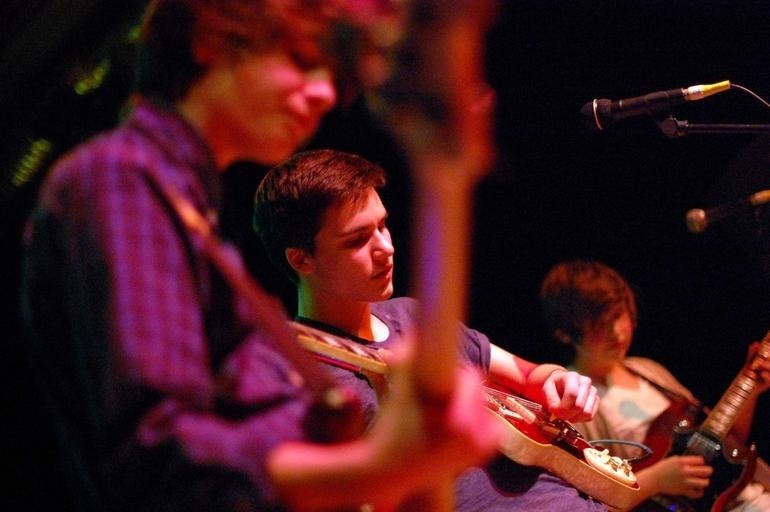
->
[247,150,623,511]
[539,260,770,512]
[2,0,509,512]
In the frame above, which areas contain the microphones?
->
[687,188,769,238]
[580,79,731,128]
[659,109,769,140]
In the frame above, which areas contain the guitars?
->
[262,319,642,508]
[629,335,769,511]
[354,0,493,509]
